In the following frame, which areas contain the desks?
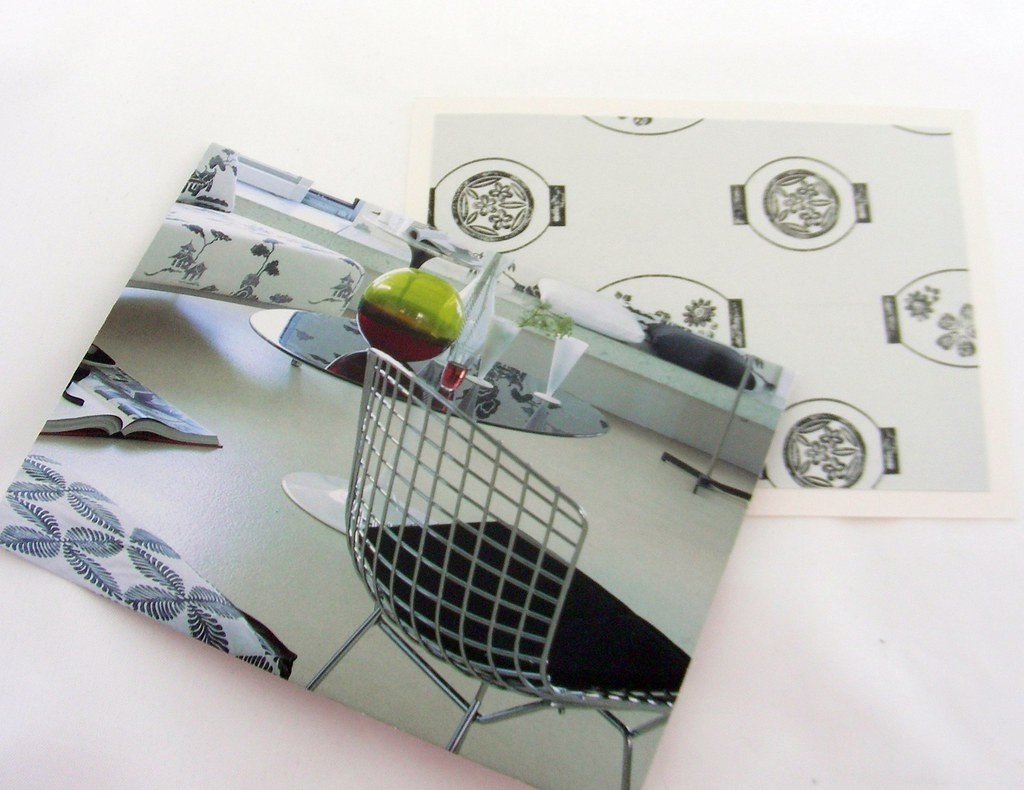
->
[249,309,611,537]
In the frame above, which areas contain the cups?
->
[440,343,477,392]
[534,332,589,404]
[466,318,520,389]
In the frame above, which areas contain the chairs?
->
[308,348,691,790]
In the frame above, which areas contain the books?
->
[39,343,224,448]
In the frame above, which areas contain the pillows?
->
[650,323,756,392]
[539,279,646,345]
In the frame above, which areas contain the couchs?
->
[126,142,364,316]
